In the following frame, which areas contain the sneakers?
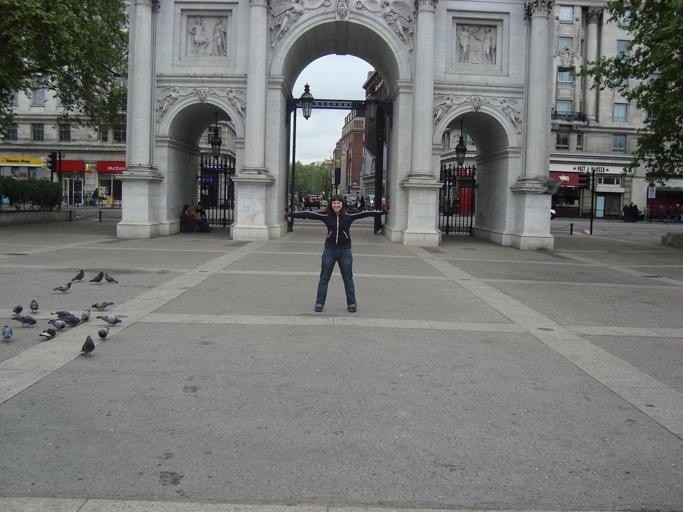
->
[315,307,322,312]
[348,307,356,312]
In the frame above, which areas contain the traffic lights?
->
[578,171,590,191]
[46,152,57,172]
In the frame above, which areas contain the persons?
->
[64,187,111,207]
[296,191,373,211]
[211,16,228,56]
[270,0,330,48]
[621,201,682,224]
[481,26,495,64]
[189,15,208,57]
[281,193,389,314]
[183,203,209,232]
[356,0,412,52]
[456,25,473,63]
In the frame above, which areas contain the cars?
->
[288,192,386,213]
[550,209,556,219]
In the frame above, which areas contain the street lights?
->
[288,82,312,225]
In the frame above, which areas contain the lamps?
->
[300,83,314,120]
[454,119,467,166]
[211,112,222,160]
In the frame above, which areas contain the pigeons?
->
[105,272,119,284]
[2,299,122,358]
[89,271,103,284]
[53,282,72,293]
[71,269,84,281]
